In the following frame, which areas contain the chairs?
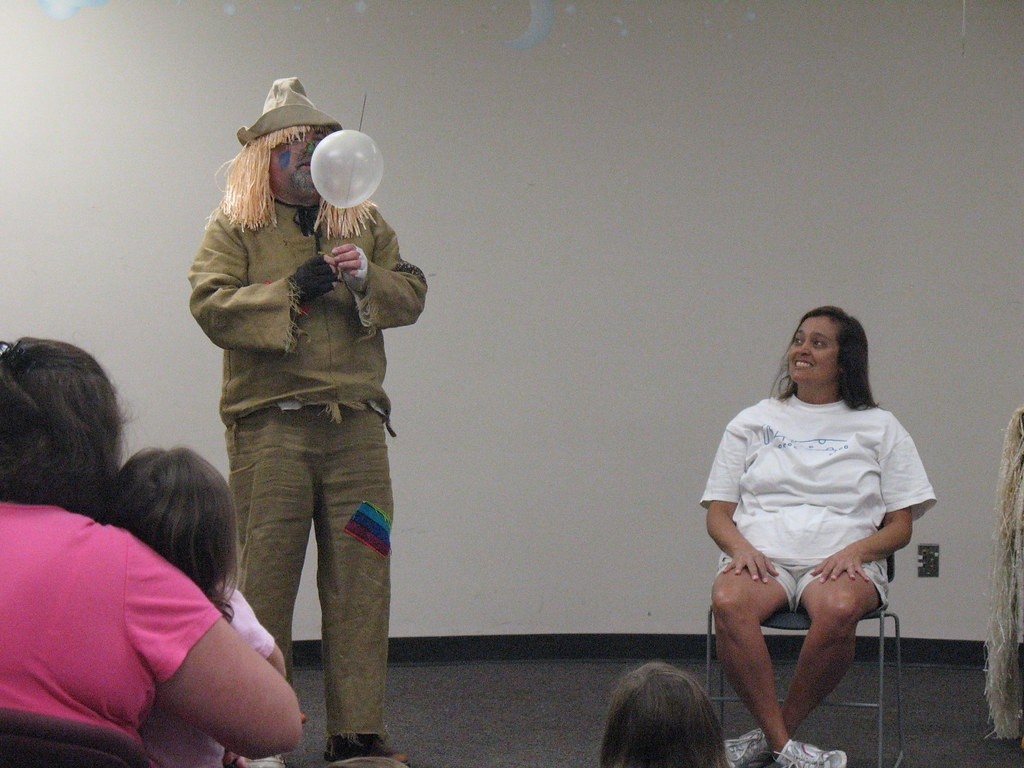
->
[707,523,904,768]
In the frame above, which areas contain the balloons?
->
[309,130,384,209]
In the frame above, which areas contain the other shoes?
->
[222,745,286,768]
[325,731,410,768]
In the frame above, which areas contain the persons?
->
[0,337,306,768]
[600,663,730,768]
[187,76,426,768]
[109,447,286,768]
[700,306,936,768]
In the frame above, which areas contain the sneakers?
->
[773,738,847,768]
[724,727,769,768]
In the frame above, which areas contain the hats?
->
[237,77,344,147]
